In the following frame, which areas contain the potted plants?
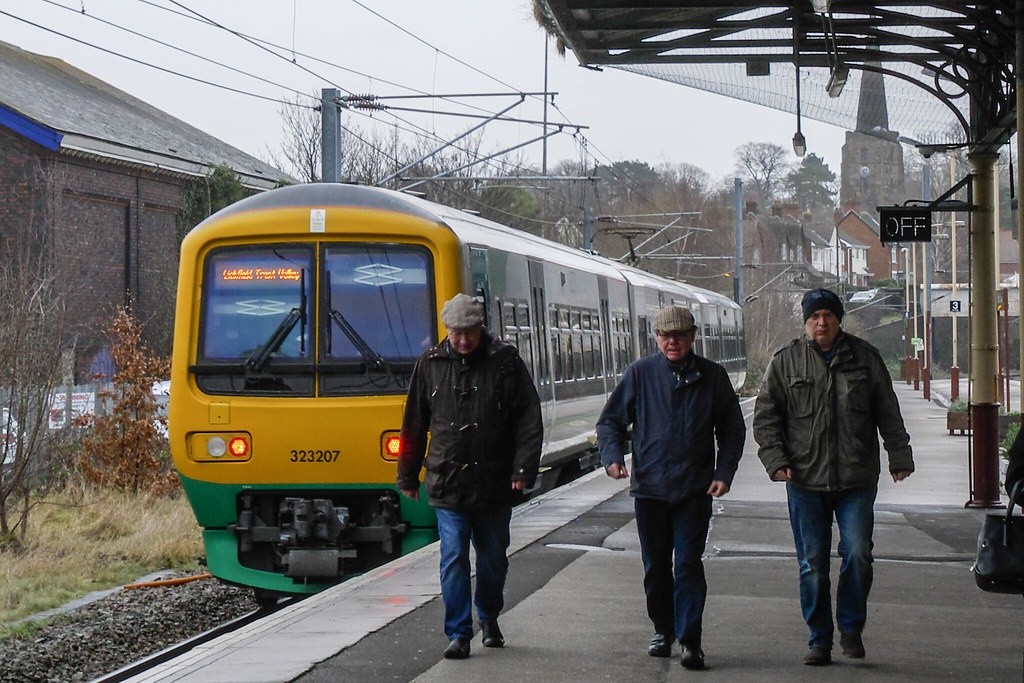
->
[946,392,1024,495]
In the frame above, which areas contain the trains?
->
[165,183,750,612]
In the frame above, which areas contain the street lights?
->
[923,221,965,402]
[913,234,949,391]
[901,247,913,384]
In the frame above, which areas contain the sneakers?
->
[647,634,671,657]
[478,620,506,648]
[443,637,471,658]
[680,644,705,668]
[838,636,865,659]
[802,647,832,665]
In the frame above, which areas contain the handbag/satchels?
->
[969,480,1024,594]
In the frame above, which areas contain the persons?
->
[596,306,748,671]
[396,292,544,658]
[752,288,916,667]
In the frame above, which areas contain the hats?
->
[801,287,845,325]
[439,292,486,334]
[651,305,695,335]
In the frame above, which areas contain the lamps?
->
[790,10,808,157]
[820,12,849,101]
